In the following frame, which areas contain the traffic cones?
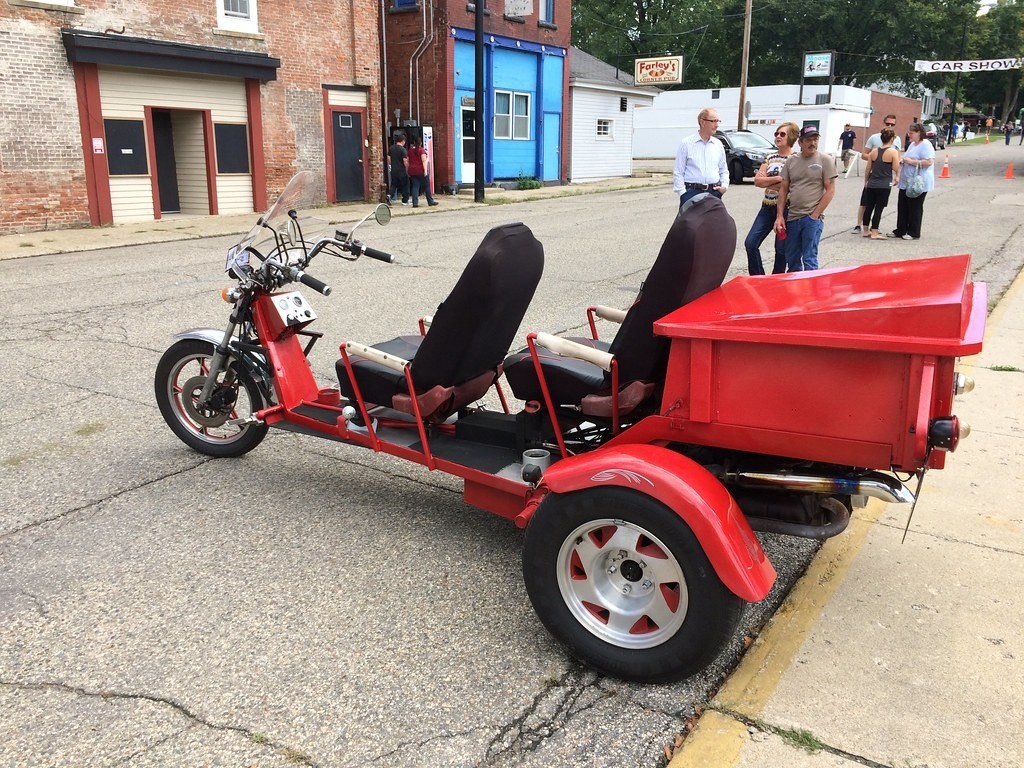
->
[1004,162,1016,179]
[938,154,951,178]
[985,133,989,144]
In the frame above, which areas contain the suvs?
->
[712,129,779,185]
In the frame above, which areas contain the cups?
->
[523,449,550,475]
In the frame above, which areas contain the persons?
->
[985,117,993,135]
[1004,121,1013,145]
[838,123,856,173]
[952,122,959,138]
[943,122,950,136]
[673,109,730,207]
[407,138,439,207]
[774,124,838,274]
[963,122,969,137]
[387,135,413,206]
[744,122,799,276]
[1018,122,1024,145]
[862,129,899,240]
[886,122,935,239]
[851,115,902,233]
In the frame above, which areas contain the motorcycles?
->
[154,171,986,683]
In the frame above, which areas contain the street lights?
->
[947,4,998,145]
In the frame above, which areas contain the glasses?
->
[884,122,895,127]
[774,131,789,137]
[702,118,721,123]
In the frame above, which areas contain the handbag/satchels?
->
[906,160,925,198]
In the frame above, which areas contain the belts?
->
[686,181,721,190]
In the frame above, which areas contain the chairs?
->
[500,194,734,414]
[334,224,546,413]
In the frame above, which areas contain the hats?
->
[800,124,819,137]
[845,123,850,127]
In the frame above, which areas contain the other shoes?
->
[878,227,882,234]
[842,168,849,173]
[851,225,861,234]
[386,195,392,206]
[902,234,915,240]
[429,202,439,206]
[413,204,418,207]
[886,230,896,238]
[402,202,411,206]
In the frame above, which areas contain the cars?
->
[905,123,945,150]
[954,121,970,132]
[961,114,985,126]
